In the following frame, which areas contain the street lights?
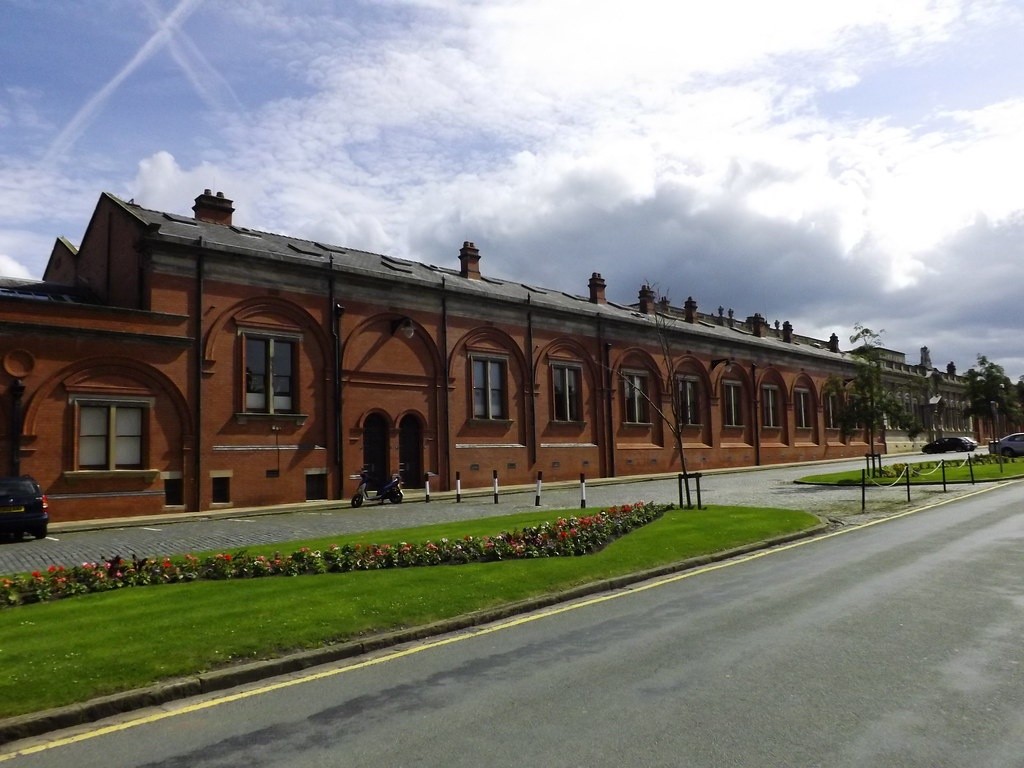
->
[990,401,1003,473]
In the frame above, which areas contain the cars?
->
[988,433,1024,457]
[957,437,978,451]
[922,438,971,454]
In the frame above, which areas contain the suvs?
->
[0,474,49,543]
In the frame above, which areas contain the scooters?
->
[351,466,412,508]
